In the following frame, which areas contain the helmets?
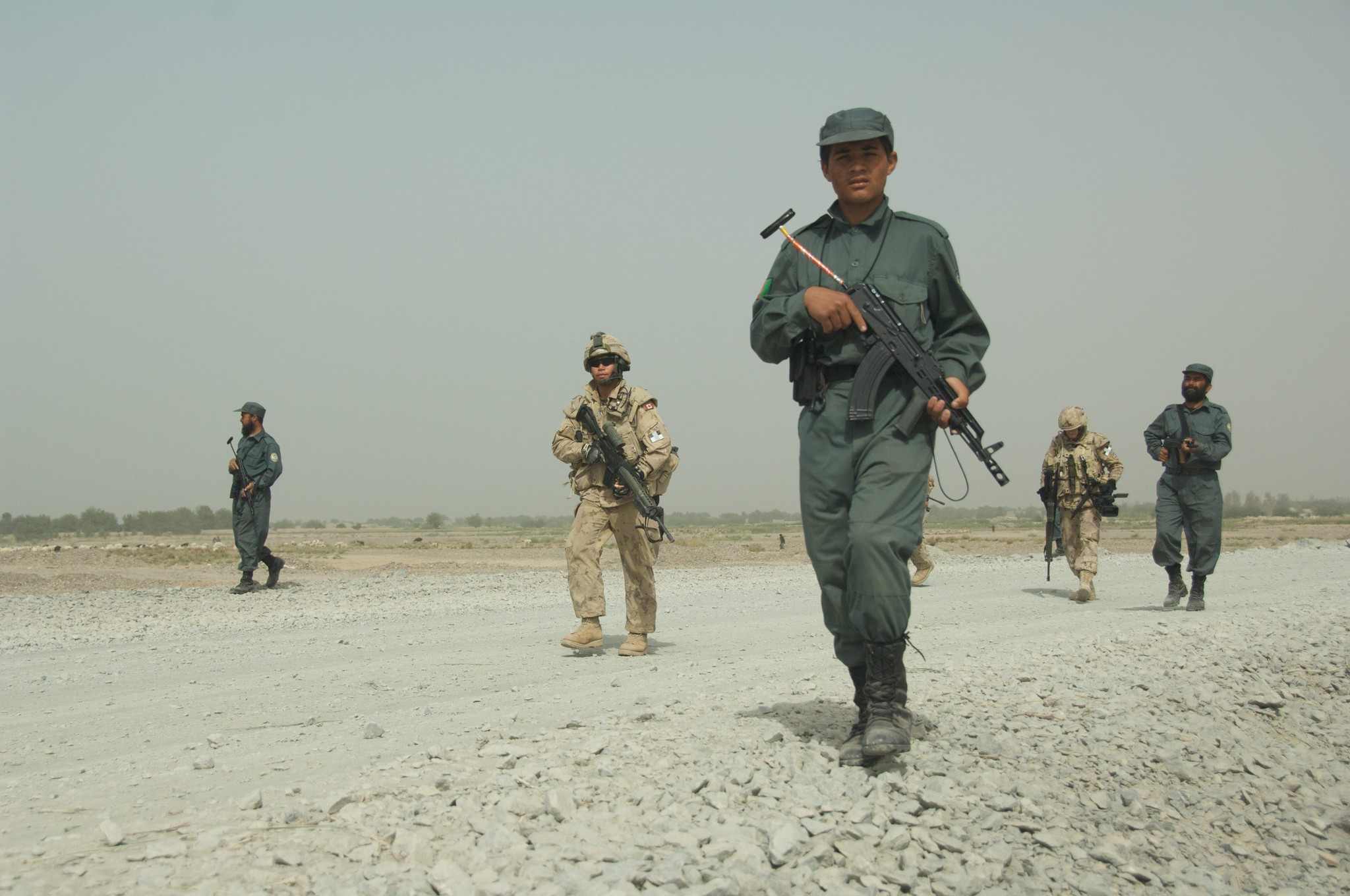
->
[583,331,631,372]
[1058,405,1088,431]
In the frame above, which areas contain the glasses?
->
[590,357,614,367]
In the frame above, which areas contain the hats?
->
[1182,363,1213,381]
[233,402,266,419]
[816,107,895,150]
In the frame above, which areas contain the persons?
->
[911,476,935,587]
[748,111,988,761]
[779,534,785,549]
[1039,408,1123,602]
[229,403,285,594]
[551,340,670,657]
[1144,363,1232,611]
[992,524,995,532]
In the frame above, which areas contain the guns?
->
[788,281,1011,488]
[1042,466,1058,582]
[1161,437,1199,467]
[227,436,255,516]
[575,403,678,544]
[925,494,945,512]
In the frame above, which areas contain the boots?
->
[1076,570,1094,602]
[618,632,649,657]
[862,640,913,756]
[229,570,254,594]
[561,616,603,650]
[1068,573,1096,601]
[838,666,881,766]
[263,554,286,588]
[1051,538,1065,557]
[1163,564,1188,607]
[1186,575,1207,611]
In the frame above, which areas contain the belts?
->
[1165,466,1215,475]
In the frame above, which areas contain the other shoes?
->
[911,558,935,585]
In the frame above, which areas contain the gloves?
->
[1104,480,1116,495]
[1037,487,1048,510]
